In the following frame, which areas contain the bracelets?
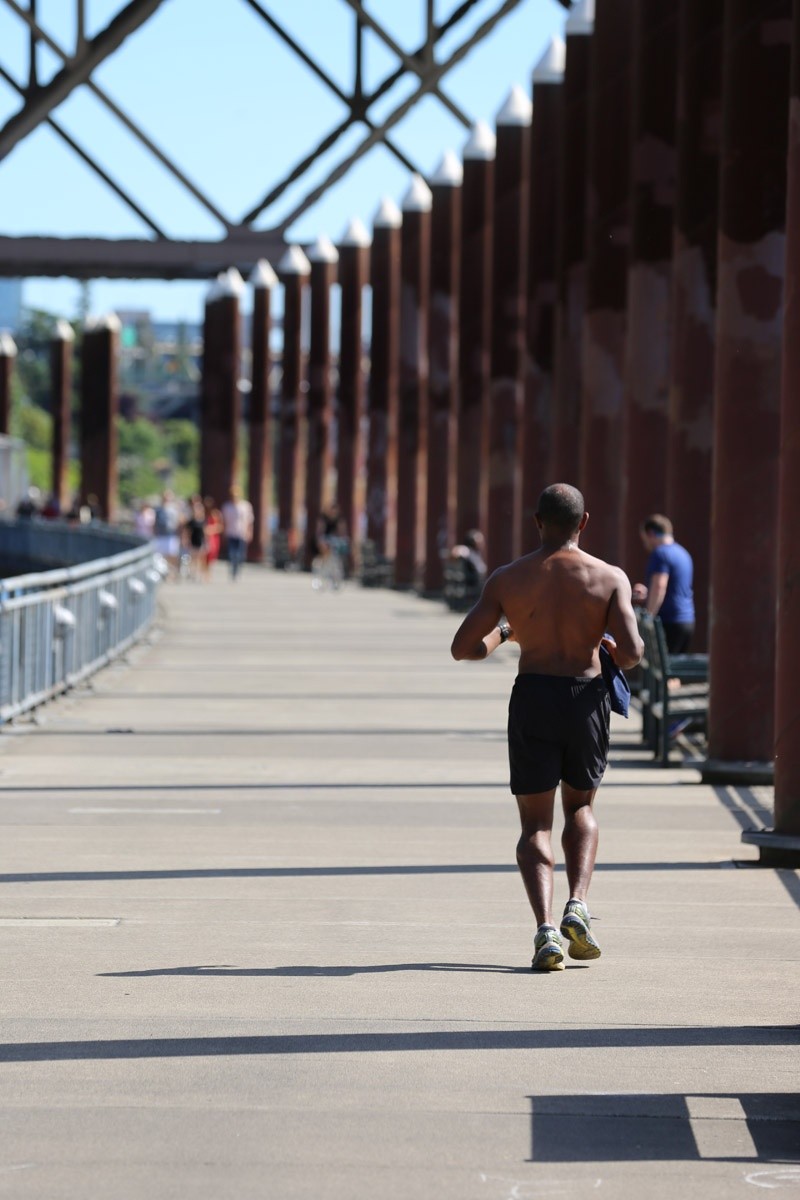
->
[497,623,510,644]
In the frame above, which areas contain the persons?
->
[634,514,696,742]
[451,482,645,973]
[222,485,254,585]
[136,487,222,581]
[318,497,348,587]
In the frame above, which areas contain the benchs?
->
[636,606,708,765]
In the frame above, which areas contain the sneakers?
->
[560,900,601,961]
[532,925,566,970]
